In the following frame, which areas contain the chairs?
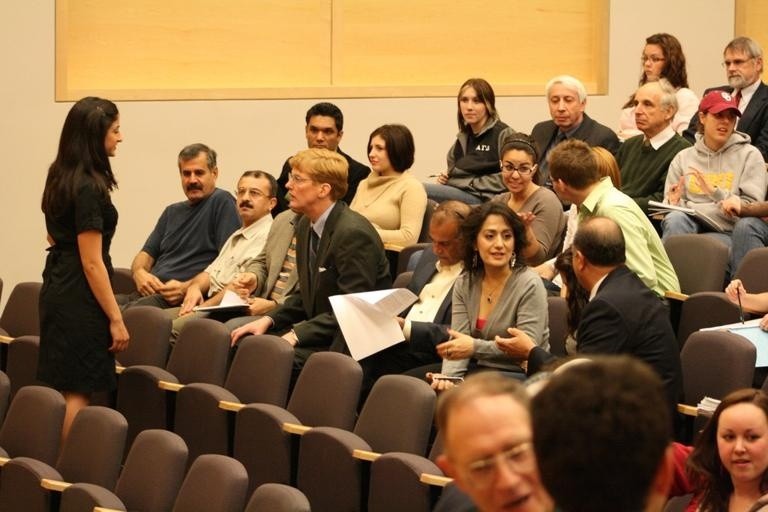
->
[1,193,768,512]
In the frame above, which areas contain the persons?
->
[436,356,768,512]
[115,34,768,425]
[35,96,131,465]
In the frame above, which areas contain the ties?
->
[734,91,742,107]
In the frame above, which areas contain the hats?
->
[698,90,743,119]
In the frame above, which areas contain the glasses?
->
[233,187,274,199]
[446,440,538,490]
[639,54,666,65]
[721,57,753,69]
[499,159,538,175]
[287,172,325,185]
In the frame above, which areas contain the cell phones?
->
[432,376,465,388]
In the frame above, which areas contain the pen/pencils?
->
[737,288,744,324]
